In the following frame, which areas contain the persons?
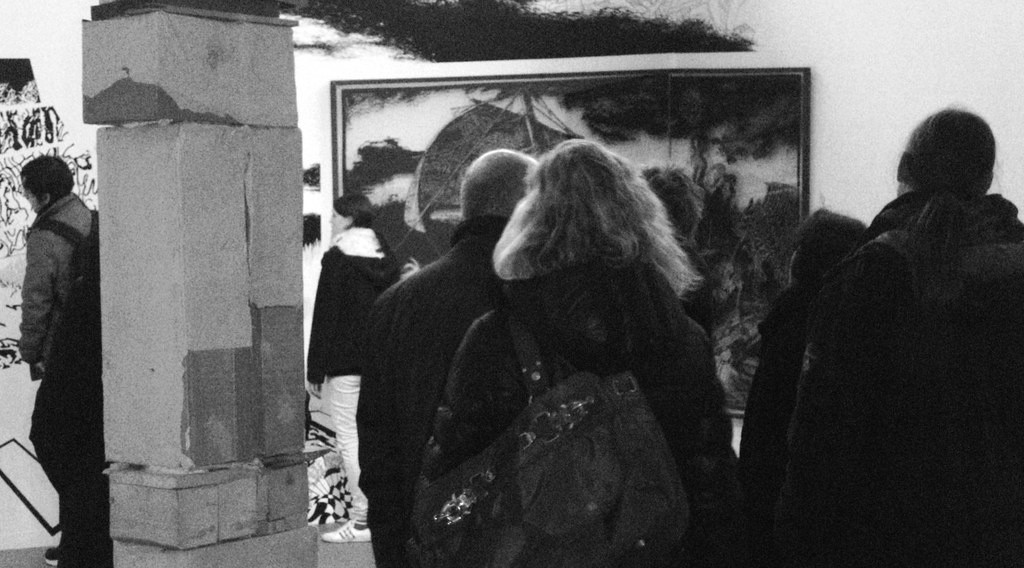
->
[16,152,117,568]
[735,202,894,566]
[308,191,401,546]
[396,138,746,567]
[636,154,716,338]
[354,148,546,568]
[780,104,1024,567]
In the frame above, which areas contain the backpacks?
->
[406,311,687,568]
[29,211,99,346]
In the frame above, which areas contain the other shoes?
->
[45,546,58,559]
